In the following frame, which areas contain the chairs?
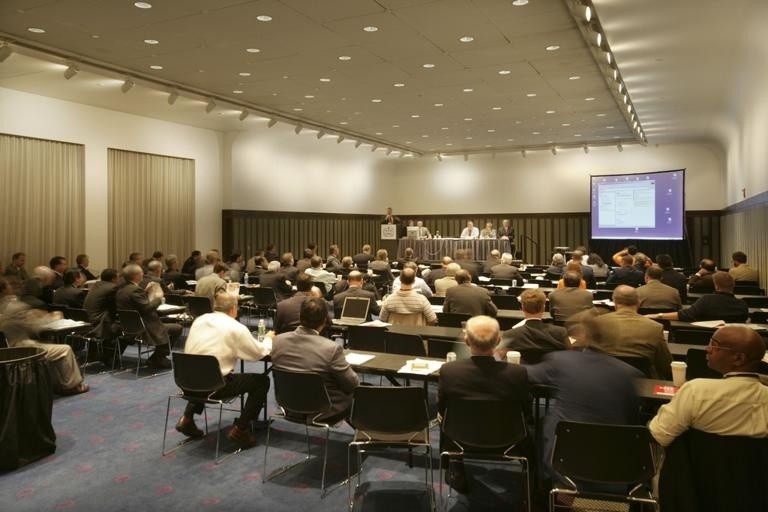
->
[751,311,767,324]
[545,274,563,279]
[685,348,725,378]
[261,366,351,498]
[310,281,327,294]
[491,295,520,311]
[347,325,385,351]
[161,351,246,465]
[749,311,768,323]
[438,396,532,512]
[110,307,174,378]
[584,277,597,287]
[736,280,761,290]
[637,307,675,316]
[640,307,674,316]
[247,287,275,333]
[429,264,441,269]
[179,294,211,322]
[62,307,105,376]
[596,276,608,282]
[508,286,525,295]
[528,279,553,288]
[594,291,613,300]
[435,312,472,328]
[515,345,558,364]
[385,332,427,352]
[246,276,261,285]
[740,296,766,308]
[373,269,387,275]
[428,296,445,306]
[527,267,543,273]
[614,355,655,377]
[163,293,184,306]
[426,336,468,361]
[347,383,436,512]
[673,427,768,512]
[545,420,659,511]
[529,265,548,268]
[605,281,618,289]
[490,278,514,286]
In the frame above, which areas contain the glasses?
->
[709,337,729,350]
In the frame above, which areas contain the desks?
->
[481,284,614,293]
[1,347,56,474]
[398,238,512,262]
[684,291,766,304]
[240,348,681,405]
[42,320,92,336]
[169,292,252,306]
[426,304,551,319]
[667,317,768,344]
[333,322,717,360]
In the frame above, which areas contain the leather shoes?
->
[226,425,257,445]
[175,415,203,438]
[55,383,64,394]
[148,352,170,366]
[64,384,89,394]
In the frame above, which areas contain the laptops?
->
[331,297,370,325]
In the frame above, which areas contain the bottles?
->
[512,279,516,288]
[244,273,248,285]
[258,319,265,343]
[447,352,456,363]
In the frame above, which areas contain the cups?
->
[662,330,669,344]
[671,361,687,387]
[505,351,521,364]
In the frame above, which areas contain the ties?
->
[505,227,508,235]
[420,227,422,236]
[389,215,391,221]
[469,230,471,236]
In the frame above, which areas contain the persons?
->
[22,277,46,304]
[729,251,735,268]
[49,256,66,287]
[229,240,414,270]
[728,251,758,281]
[499,219,515,252]
[271,297,359,425]
[143,251,163,263]
[633,267,681,309]
[443,269,497,317]
[648,272,748,323]
[84,269,132,355]
[656,254,688,286]
[567,246,589,266]
[165,254,183,280]
[634,252,652,270]
[116,265,182,368]
[607,255,644,285]
[195,262,229,299]
[546,253,566,275]
[524,320,643,493]
[588,285,674,380]
[605,256,644,286]
[145,260,174,291]
[6,253,30,279]
[381,207,400,225]
[430,256,456,286]
[656,253,687,291]
[461,220,479,238]
[688,259,722,289]
[557,261,586,289]
[33,266,55,300]
[439,315,534,493]
[435,263,467,295]
[379,269,438,327]
[586,253,608,277]
[274,275,321,333]
[454,249,479,282]
[76,254,97,280]
[174,293,277,446]
[404,218,414,234]
[479,222,497,239]
[205,251,220,265]
[490,252,524,281]
[416,221,431,239]
[129,253,143,266]
[53,269,86,308]
[612,246,640,267]
[549,270,593,323]
[393,262,432,298]
[333,271,381,322]
[0,276,89,396]
[500,289,571,365]
[642,323,768,460]
[180,251,204,273]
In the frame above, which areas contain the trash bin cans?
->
[0,345,57,476]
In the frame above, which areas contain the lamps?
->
[573,5,590,22]
[436,155,442,161]
[317,131,325,139]
[239,110,250,121]
[206,101,217,113]
[587,31,602,48]
[583,146,589,154]
[463,154,468,162]
[267,118,279,128]
[385,148,393,156]
[167,91,179,105]
[63,65,80,80]
[521,149,527,158]
[121,79,135,94]
[607,68,617,80]
[355,140,362,148]
[613,83,622,92]
[370,144,378,152]
[617,144,623,153]
[294,124,303,135]
[0,45,13,63]
[551,148,557,156]
[337,135,344,143]
[601,52,611,65]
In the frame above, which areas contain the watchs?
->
[657,313,663,318]
